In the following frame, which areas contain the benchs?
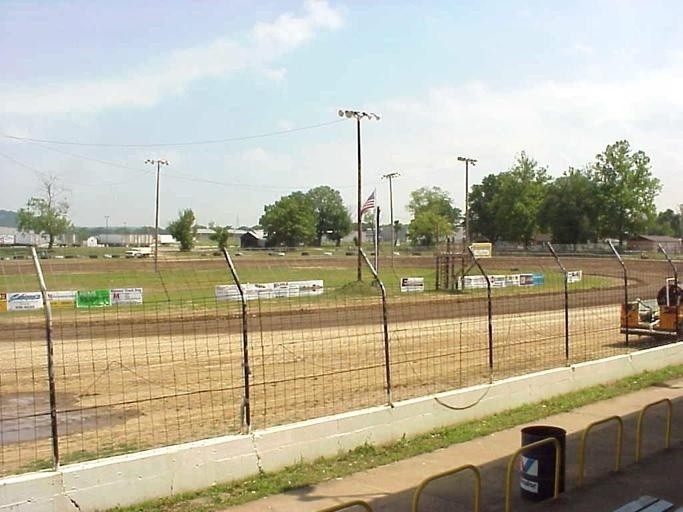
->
[614,493,682,512]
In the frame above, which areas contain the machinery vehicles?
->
[621,283,683,339]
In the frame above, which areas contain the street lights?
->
[146,159,169,274]
[105,216,109,244]
[340,112,380,281]
[457,158,477,260]
[382,172,401,267]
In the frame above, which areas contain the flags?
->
[361,191,375,215]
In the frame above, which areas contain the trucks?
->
[125,247,151,258]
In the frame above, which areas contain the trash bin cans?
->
[521,426,565,499]
